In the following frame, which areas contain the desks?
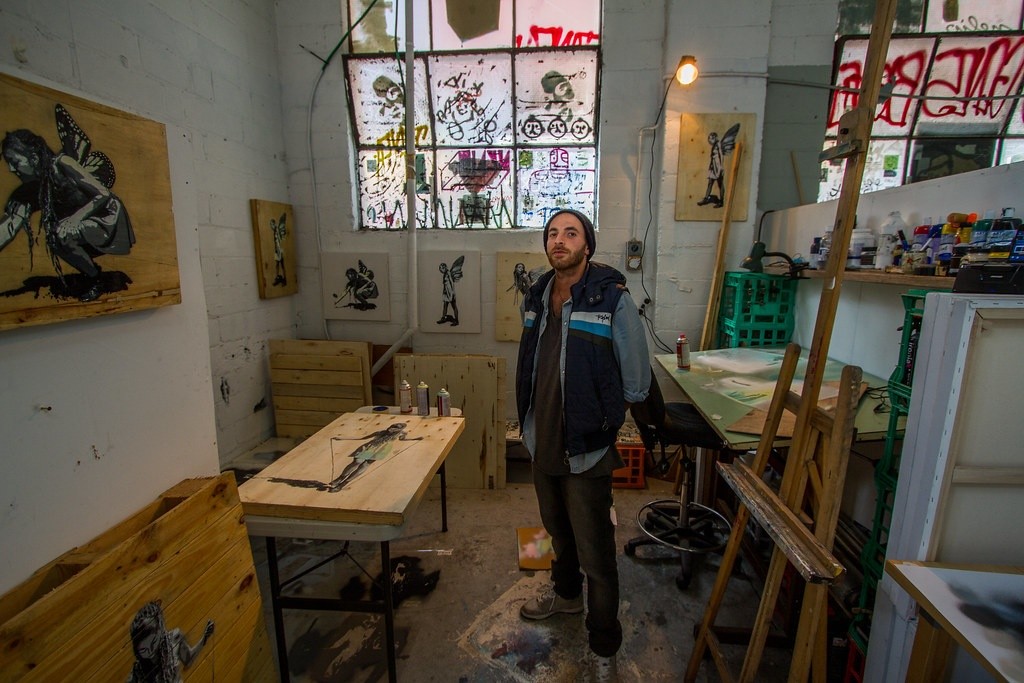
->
[654,343,906,507]
[237,412,466,683]
[884,559,1023,683]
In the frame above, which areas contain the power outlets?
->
[628,241,642,256]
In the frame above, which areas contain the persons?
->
[515,209,666,683]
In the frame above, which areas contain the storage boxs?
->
[612,444,646,489]
[716,271,797,349]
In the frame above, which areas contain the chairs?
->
[624,365,733,591]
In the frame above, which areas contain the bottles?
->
[818,226,834,270]
[845,229,875,268]
[809,237,822,270]
[399,380,413,413]
[874,211,906,268]
[438,388,450,416]
[417,382,430,416]
[676,334,690,370]
[911,207,1024,267]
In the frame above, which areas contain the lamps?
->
[638,54,699,297]
[738,242,810,280]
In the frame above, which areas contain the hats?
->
[543,208,596,260]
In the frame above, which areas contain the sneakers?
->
[520,588,584,619]
[590,651,618,683]
